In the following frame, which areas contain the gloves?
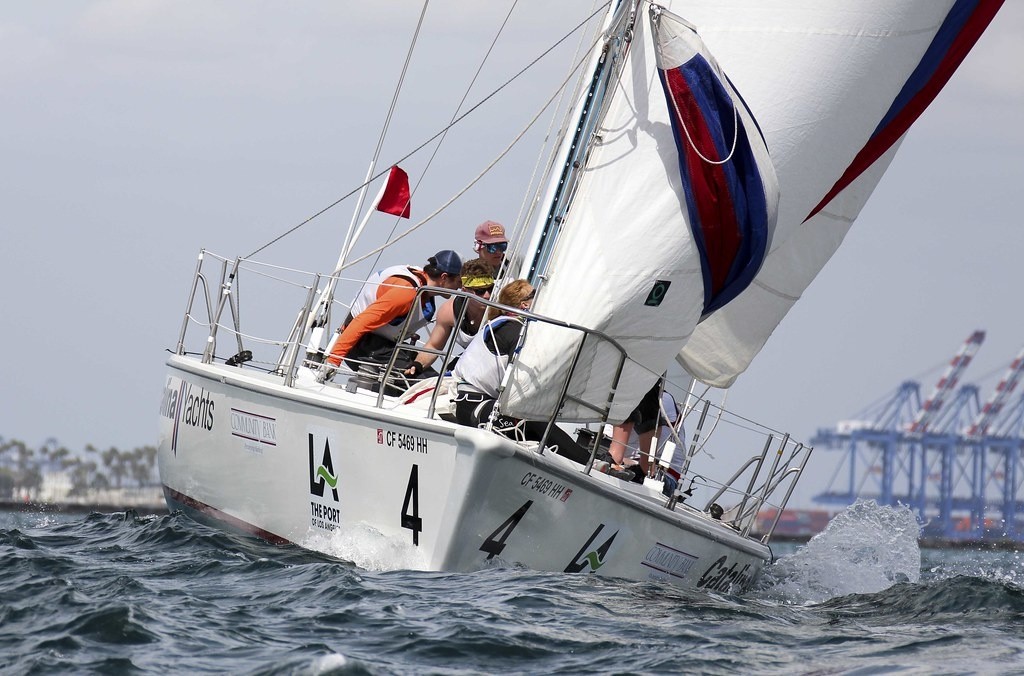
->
[316,363,338,385]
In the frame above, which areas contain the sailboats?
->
[154,1,1003,598]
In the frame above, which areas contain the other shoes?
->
[592,451,646,484]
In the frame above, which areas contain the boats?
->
[744,326,1023,554]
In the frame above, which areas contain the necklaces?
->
[470,319,475,325]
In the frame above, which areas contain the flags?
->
[374,165,410,219]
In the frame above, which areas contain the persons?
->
[316,249,463,398]
[450,279,687,497]
[403,258,495,377]
[472,221,510,280]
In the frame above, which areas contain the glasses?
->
[521,289,537,301]
[465,284,493,296]
[482,242,507,254]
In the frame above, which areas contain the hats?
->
[475,220,508,244]
[431,250,462,274]
